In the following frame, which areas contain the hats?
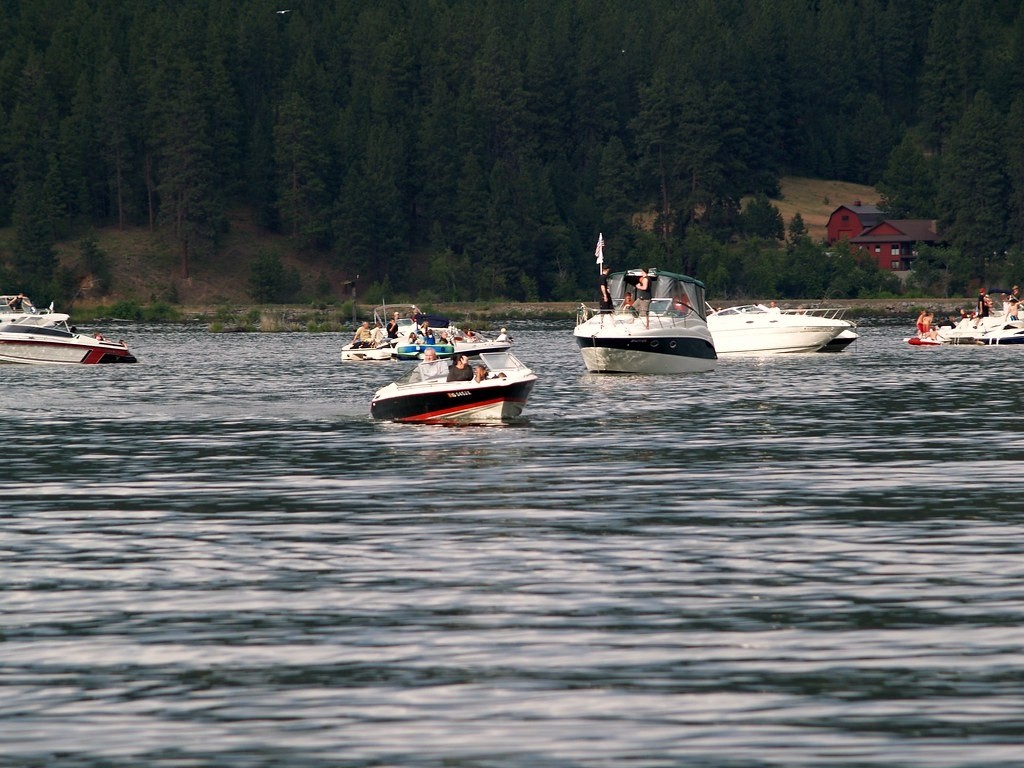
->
[978,288,986,293]
[1012,285,1018,290]
[394,312,399,314]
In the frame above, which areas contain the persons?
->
[705,306,723,315]
[768,301,823,317]
[95,330,126,345]
[597,263,617,330]
[387,311,401,348]
[446,354,474,382]
[916,284,1024,343]
[9,293,33,310]
[407,306,481,345]
[408,347,449,383]
[350,321,383,349]
[578,302,588,325]
[626,266,652,329]
[474,366,507,383]
[618,293,634,313]
[495,328,515,343]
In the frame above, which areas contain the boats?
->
[0,294,139,368]
[707,305,850,354]
[339,313,509,364]
[571,232,718,376]
[908,285,1024,347]
[368,351,539,427]
[817,328,862,353]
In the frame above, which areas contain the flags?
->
[594,239,605,257]
